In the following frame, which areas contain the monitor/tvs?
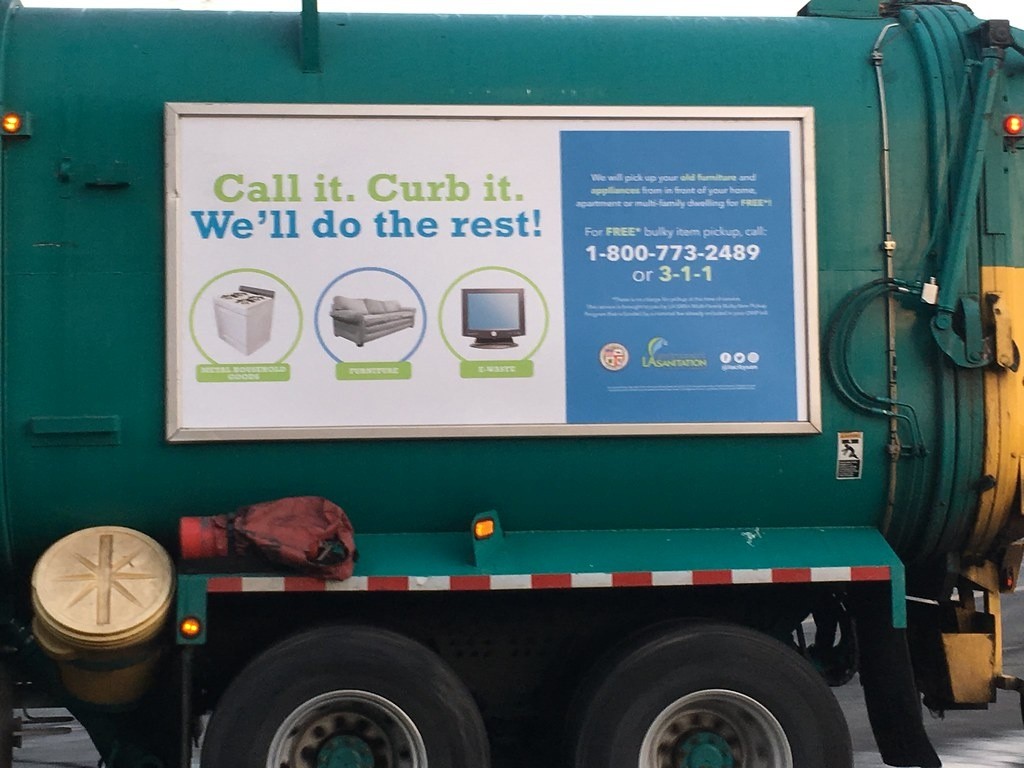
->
[461,289,526,349]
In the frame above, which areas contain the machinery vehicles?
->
[0,0,1024,768]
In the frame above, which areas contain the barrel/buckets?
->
[31,524,177,707]
